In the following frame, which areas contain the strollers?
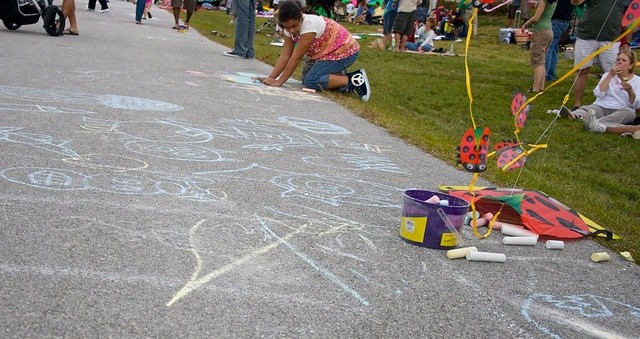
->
[0,0,66,36]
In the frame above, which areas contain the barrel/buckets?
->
[400,189,470,249]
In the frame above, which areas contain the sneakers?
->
[345,68,371,102]
[223,51,246,59]
[98,9,110,13]
[84,6,95,11]
[561,106,581,121]
[582,108,604,133]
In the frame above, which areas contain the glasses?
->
[284,21,300,29]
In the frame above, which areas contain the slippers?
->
[147,11,152,18]
[141,17,146,19]
[62,29,79,35]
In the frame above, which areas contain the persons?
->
[521,0,557,93]
[372,2,386,24]
[334,0,360,23]
[135,0,145,24]
[392,0,422,52]
[543,0,573,80]
[250,0,372,103]
[171,0,196,30]
[583,109,640,135]
[141,0,153,19]
[84,0,110,13]
[406,17,437,54]
[223,0,256,60]
[571,0,632,111]
[559,48,640,125]
[382,0,400,49]
[507,0,521,28]
[42,0,80,36]
[354,4,373,25]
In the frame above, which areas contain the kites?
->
[437,182,623,242]
[621,0,640,32]
[454,126,491,173]
[510,87,531,128]
[493,137,529,171]
